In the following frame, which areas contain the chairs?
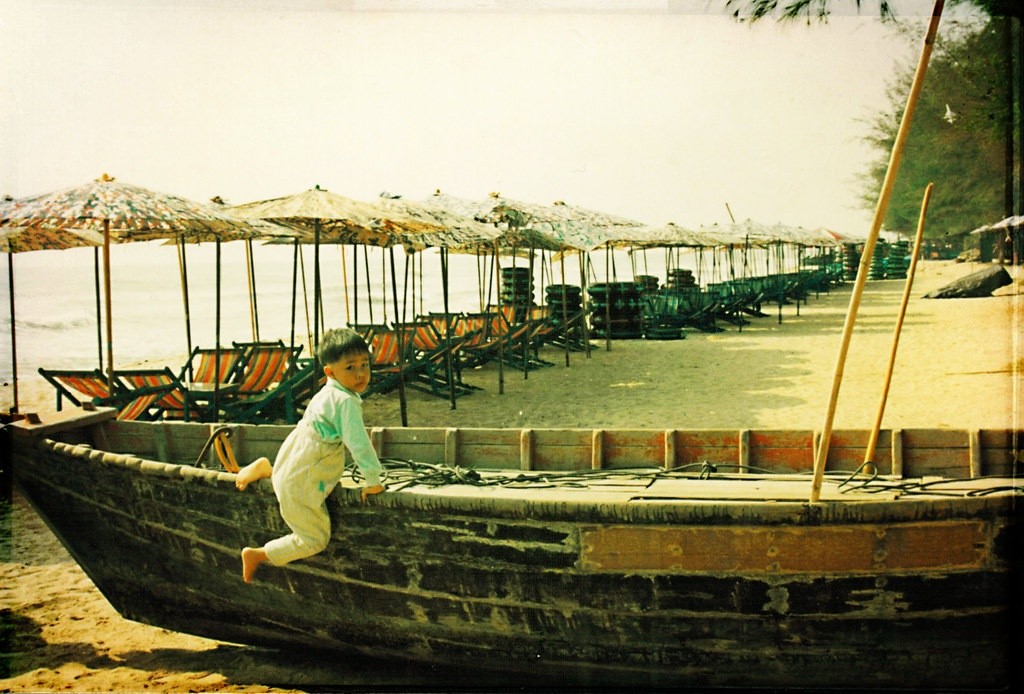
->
[37,305,556,423]
[642,269,850,333]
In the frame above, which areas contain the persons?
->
[236,328,387,583]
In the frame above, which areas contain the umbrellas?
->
[0,173,867,428]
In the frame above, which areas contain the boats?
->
[0,0,1024,694]
